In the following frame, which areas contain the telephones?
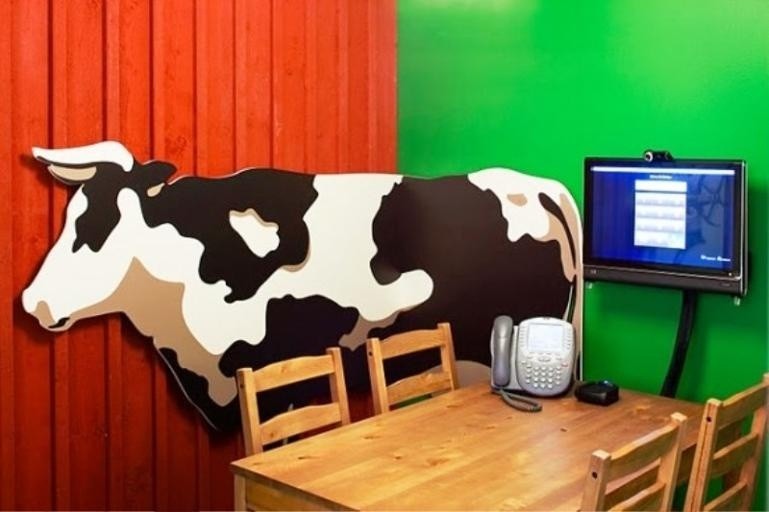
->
[490,316,574,397]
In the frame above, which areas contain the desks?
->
[228,379,746,512]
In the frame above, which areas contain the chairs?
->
[683,372,769,512]
[580,411,689,512]
[235,345,352,458]
[366,322,461,415]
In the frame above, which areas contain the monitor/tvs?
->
[583,158,747,298]
